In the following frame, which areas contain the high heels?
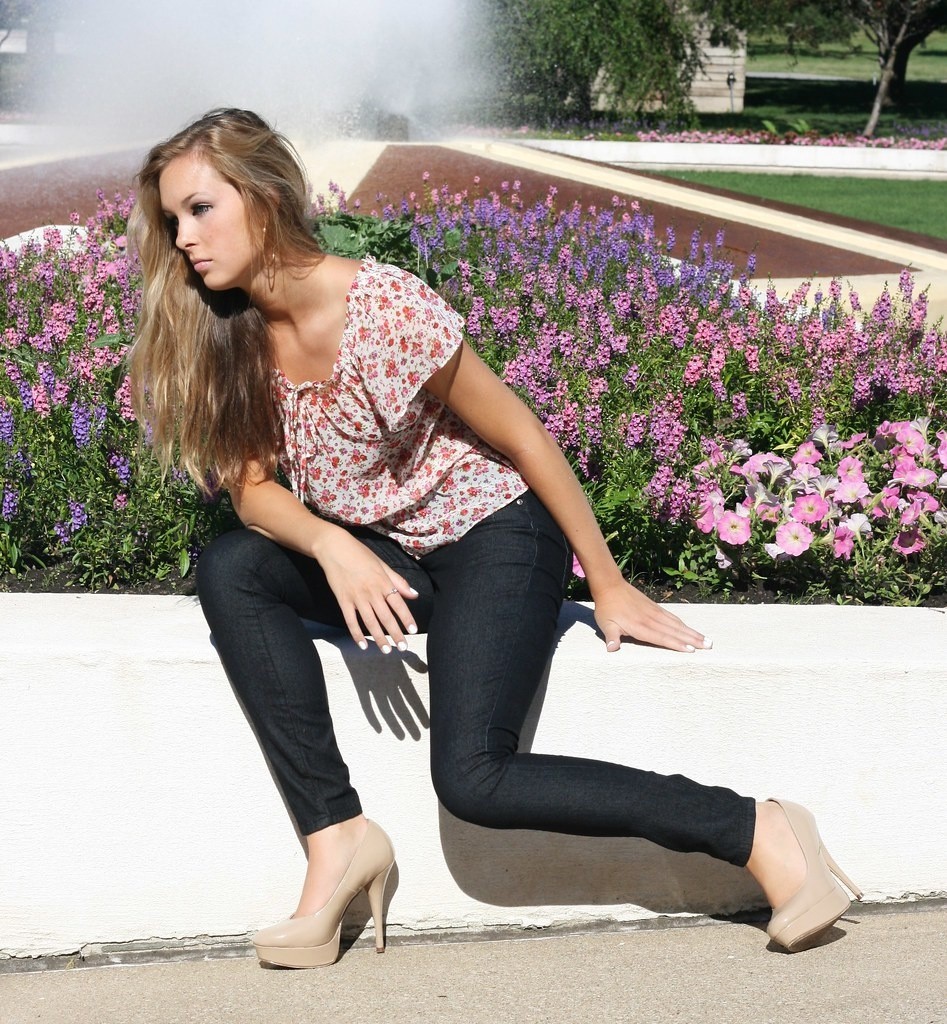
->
[254,819,395,969]
[765,799,863,951]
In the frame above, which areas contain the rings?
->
[384,587,401,599]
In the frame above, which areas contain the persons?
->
[118,108,864,973]
